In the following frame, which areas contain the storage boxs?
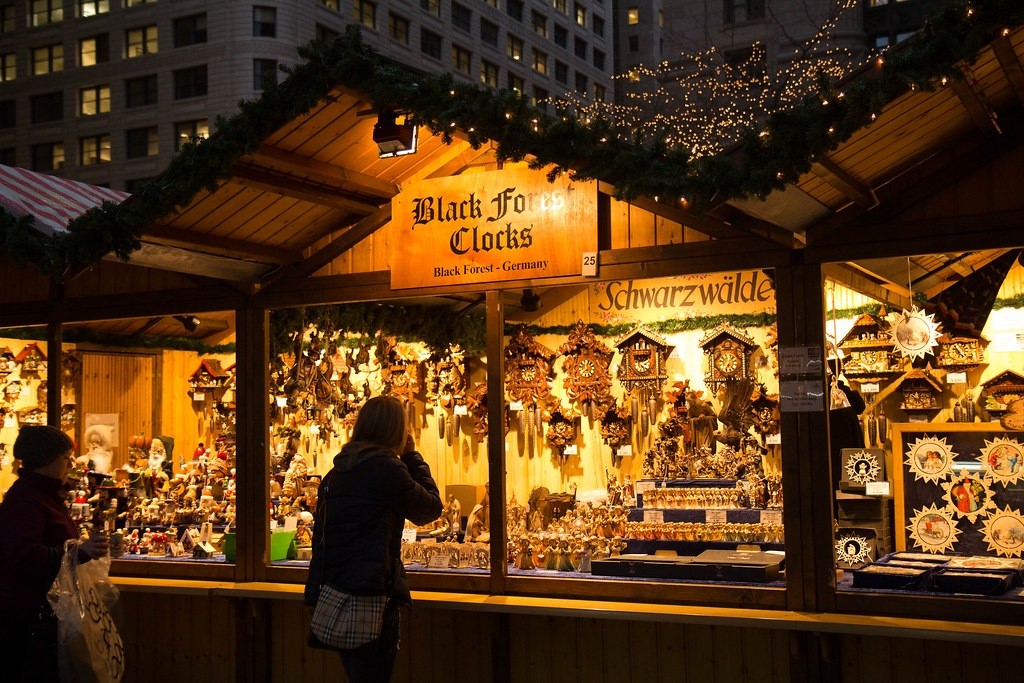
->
[224,527,298,562]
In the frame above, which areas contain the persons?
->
[0,425,109,683]
[301,395,442,682]
[70,424,236,556]
[269,346,786,573]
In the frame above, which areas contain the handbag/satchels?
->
[307,581,391,651]
[46,538,125,683]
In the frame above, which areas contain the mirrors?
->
[885,304,944,363]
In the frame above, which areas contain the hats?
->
[14,424,74,470]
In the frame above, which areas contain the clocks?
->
[634,356,651,373]
[523,365,537,383]
[859,348,878,366]
[947,342,971,362]
[610,421,620,435]
[392,371,408,387]
[555,421,567,435]
[719,351,738,373]
[578,359,597,379]
[441,367,455,384]
[911,391,927,405]
[758,406,774,423]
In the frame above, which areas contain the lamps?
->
[374,107,421,160]
[519,287,544,312]
[174,312,199,333]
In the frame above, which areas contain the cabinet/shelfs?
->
[892,421,1024,560]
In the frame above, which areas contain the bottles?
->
[76,491,86,503]
[194,443,206,460]
